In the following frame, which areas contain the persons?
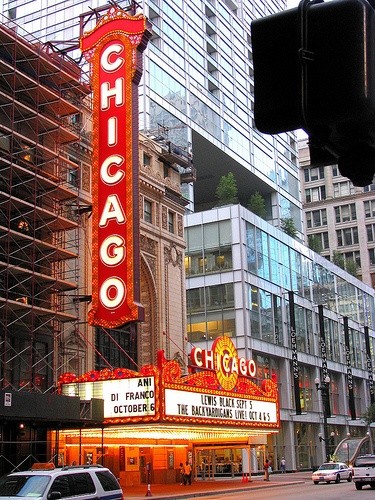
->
[178,461,192,486]
[280,457,286,473]
[264,460,270,481]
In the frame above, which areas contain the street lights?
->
[315,377,335,461]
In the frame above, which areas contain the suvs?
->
[0,461,125,500]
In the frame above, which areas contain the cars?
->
[351,453,375,491]
[312,460,354,485]
